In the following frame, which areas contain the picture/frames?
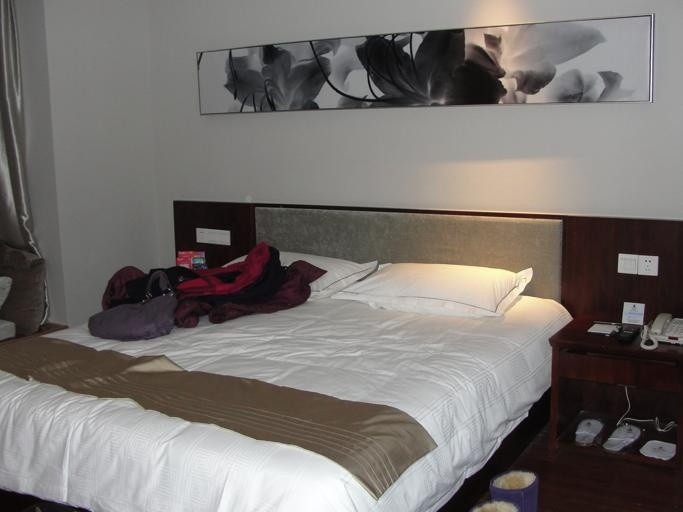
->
[194,14,654,117]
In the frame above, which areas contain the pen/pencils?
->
[593,320,618,325]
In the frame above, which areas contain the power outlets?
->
[637,254,659,277]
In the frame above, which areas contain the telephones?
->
[649,313,683,346]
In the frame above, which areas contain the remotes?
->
[617,325,641,342]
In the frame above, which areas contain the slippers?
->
[603,425,640,450]
[575,419,603,448]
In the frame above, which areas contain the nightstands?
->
[548,317,683,470]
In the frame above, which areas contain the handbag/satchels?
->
[89,270,178,341]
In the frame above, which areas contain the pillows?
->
[0,243,47,334]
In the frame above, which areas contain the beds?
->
[0,205,565,512]
